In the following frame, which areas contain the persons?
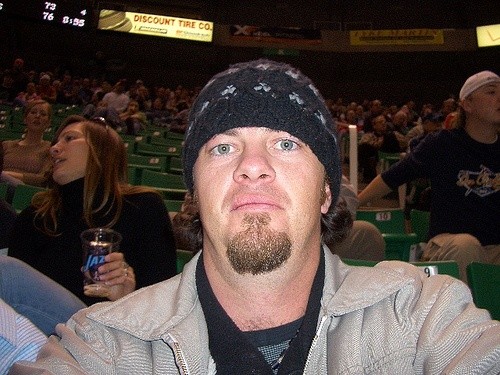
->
[329,175,386,263]
[0,114,178,341]
[9,58,500,374]
[0,298,51,375]
[1,55,470,201]
[355,70,500,286]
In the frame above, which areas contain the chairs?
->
[0,101,500,322]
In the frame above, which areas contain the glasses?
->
[91,116,109,159]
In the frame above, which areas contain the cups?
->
[80,228,122,297]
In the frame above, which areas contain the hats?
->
[183,58,341,203]
[460,71,500,103]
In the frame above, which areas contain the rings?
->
[119,260,128,269]
[121,268,128,276]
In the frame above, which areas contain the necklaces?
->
[268,317,303,370]
